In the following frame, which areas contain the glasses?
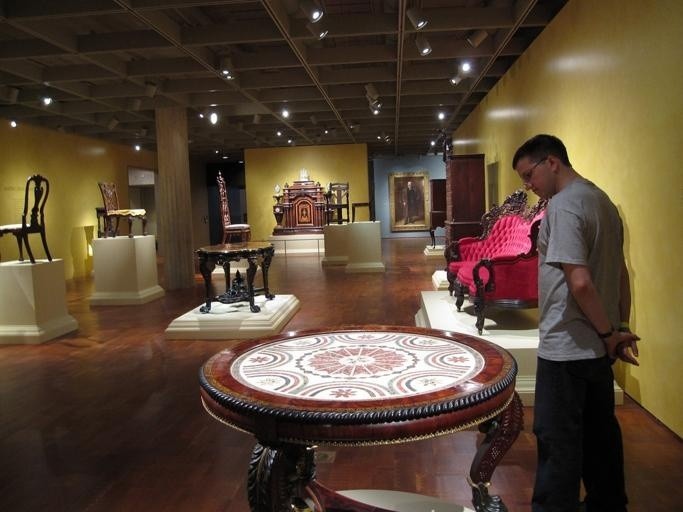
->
[522,157,548,183]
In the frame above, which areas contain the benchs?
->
[442,189,548,334]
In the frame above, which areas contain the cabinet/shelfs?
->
[428,152,485,250]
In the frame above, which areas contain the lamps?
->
[298,1,328,42]
[103,81,158,132]
[403,1,489,89]
[363,83,384,116]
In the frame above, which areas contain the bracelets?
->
[619,322,630,329]
[599,325,615,339]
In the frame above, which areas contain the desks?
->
[198,323,525,511]
[196,242,274,314]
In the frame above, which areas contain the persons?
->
[512,133,640,512]
[401,181,420,225]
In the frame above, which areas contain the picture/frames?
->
[388,171,431,233]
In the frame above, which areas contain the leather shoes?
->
[579,500,627,512]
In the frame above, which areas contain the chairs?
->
[216,170,252,247]
[0,174,53,264]
[322,181,376,226]
[93,180,146,238]
